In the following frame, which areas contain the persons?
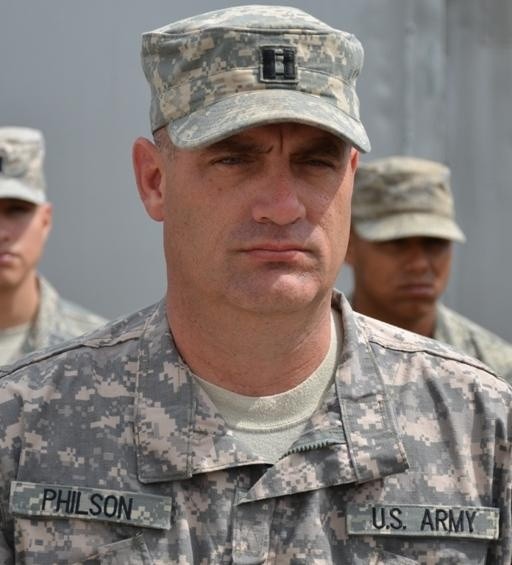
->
[342,157,511,386]
[0,4,511,564]
[0,124,113,366]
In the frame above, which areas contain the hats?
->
[351,156,467,244]
[0,125,46,205]
[141,4,371,153]
